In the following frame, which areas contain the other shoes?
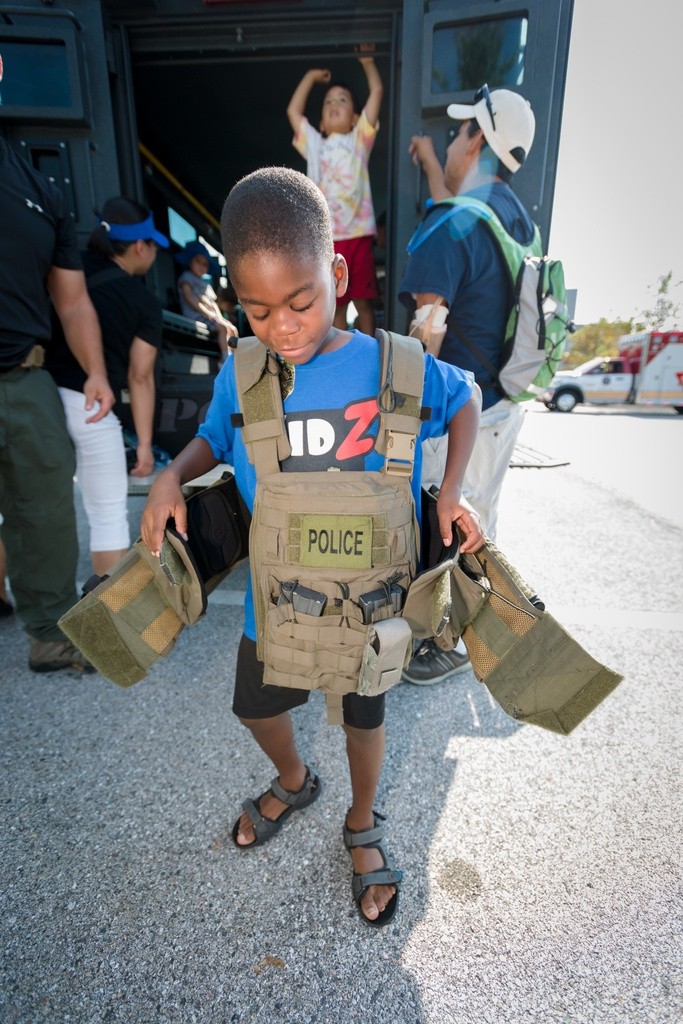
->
[218,358,225,370]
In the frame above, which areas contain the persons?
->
[0,195,168,618]
[399,88,535,684]
[287,42,383,336]
[141,166,484,925]
[608,362,615,373]
[1,141,118,673]
[175,242,239,369]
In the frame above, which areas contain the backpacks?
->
[425,197,570,404]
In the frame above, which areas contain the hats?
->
[447,88,536,174]
[105,212,169,247]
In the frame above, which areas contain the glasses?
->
[476,83,497,133]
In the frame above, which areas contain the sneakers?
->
[403,636,472,685]
[26,635,95,673]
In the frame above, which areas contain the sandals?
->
[342,808,403,926]
[232,766,321,849]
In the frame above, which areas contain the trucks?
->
[537,329,683,413]
